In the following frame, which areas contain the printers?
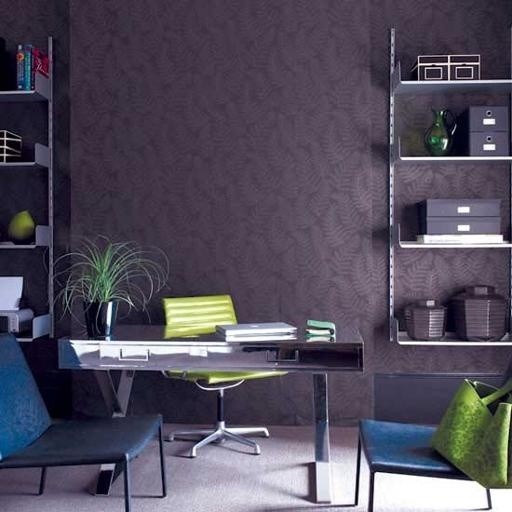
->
[0,275,34,336]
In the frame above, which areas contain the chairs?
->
[159,294,270,459]
[354,362,512,512]
[0,333,166,512]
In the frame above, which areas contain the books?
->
[32,47,50,91]
[303,319,335,336]
[17,44,25,90]
[24,45,32,92]
[305,333,338,343]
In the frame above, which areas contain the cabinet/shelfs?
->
[0,35,71,344]
[387,27,512,349]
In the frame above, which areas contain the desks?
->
[57,322,365,504]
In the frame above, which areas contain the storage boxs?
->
[409,54,482,82]
[457,105,510,156]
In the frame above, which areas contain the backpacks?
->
[429,378,512,489]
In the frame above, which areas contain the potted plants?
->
[52,232,171,338]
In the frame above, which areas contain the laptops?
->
[216,330,297,342]
[215,321,297,336]
